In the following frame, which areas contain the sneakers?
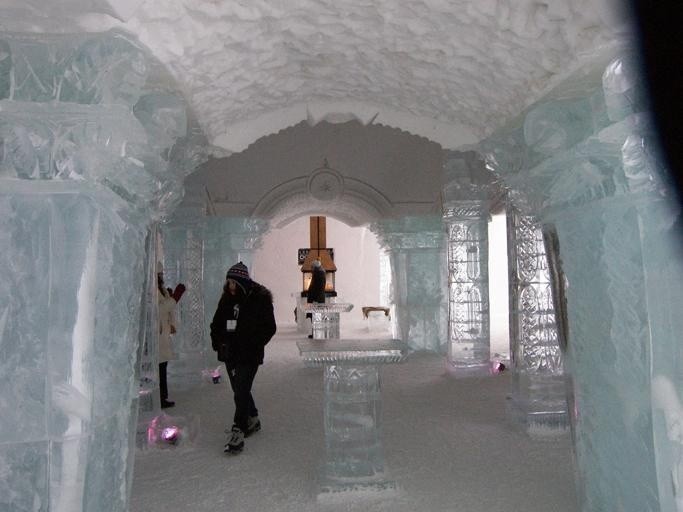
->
[161,400,175,408]
[308,334,313,338]
[222,423,246,456]
[244,414,262,439]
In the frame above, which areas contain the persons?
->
[156,260,187,410]
[209,259,276,454]
[305,257,327,339]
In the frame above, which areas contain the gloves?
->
[173,283,186,304]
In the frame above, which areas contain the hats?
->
[225,263,251,295]
[311,257,322,268]
